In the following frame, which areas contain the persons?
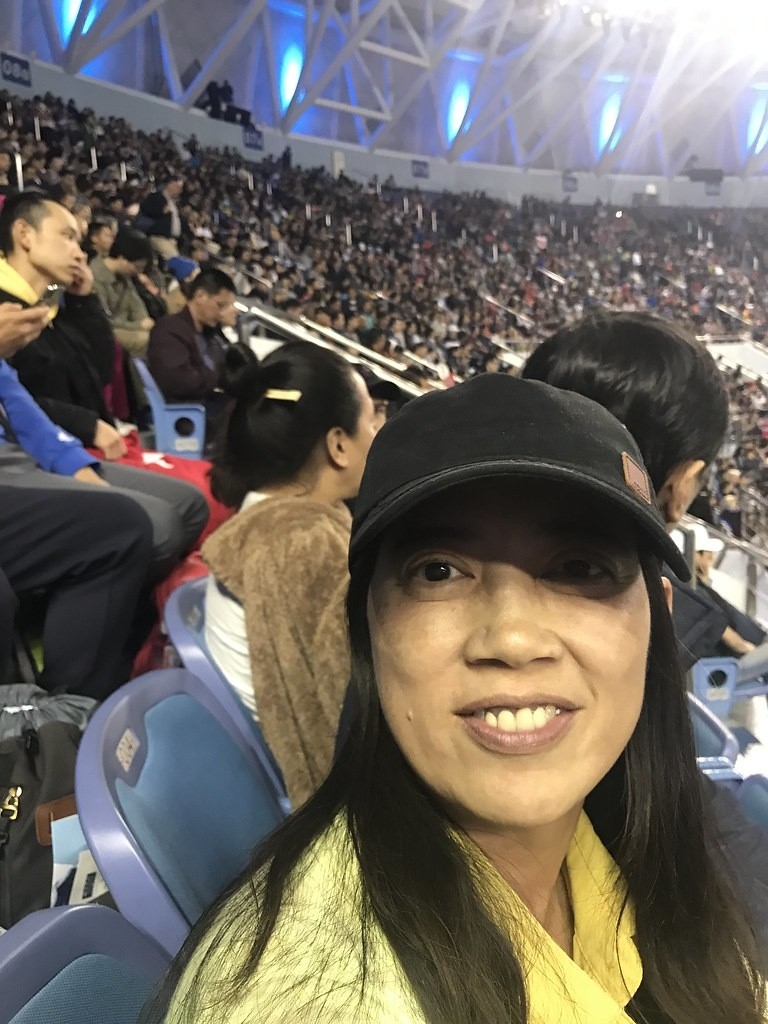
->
[200,339,376,811]
[0,90,767,700]
[141,372,767,1024]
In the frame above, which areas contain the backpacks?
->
[0,683,122,929]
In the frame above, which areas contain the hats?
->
[167,256,200,284]
[348,371,692,583]
[670,523,725,553]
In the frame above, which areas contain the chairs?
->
[690,658,768,720]
[684,690,738,766]
[133,356,206,460]
[74,667,283,959]
[162,576,290,818]
[0,903,171,1024]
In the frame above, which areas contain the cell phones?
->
[31,285,66,308]
